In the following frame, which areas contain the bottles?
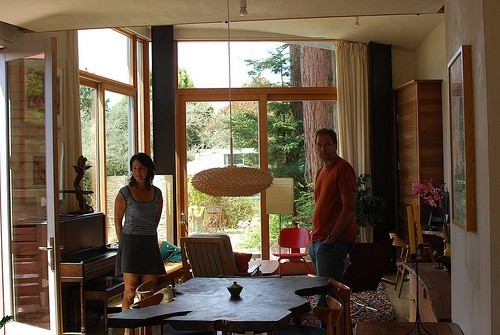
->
[181,211,186,223]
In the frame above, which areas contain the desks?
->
[107,278,330,335]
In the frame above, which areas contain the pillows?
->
[233,252,252,273]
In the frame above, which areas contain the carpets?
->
[282,276,396,328]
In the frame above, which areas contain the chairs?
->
[129,228,351,335]
[389,233,434,298]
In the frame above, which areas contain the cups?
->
[227,287,243,297]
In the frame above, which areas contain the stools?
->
[75,276,125,335]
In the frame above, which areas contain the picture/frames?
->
[21,60,64,129]
[446,44,476,231]
[20,137,64,201]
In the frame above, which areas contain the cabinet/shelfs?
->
[403,262,451,322]
[396,79,443,228]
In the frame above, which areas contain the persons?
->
[114,153,167,335]
[308,127,358,335]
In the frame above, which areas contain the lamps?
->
[191,0,273,197]
